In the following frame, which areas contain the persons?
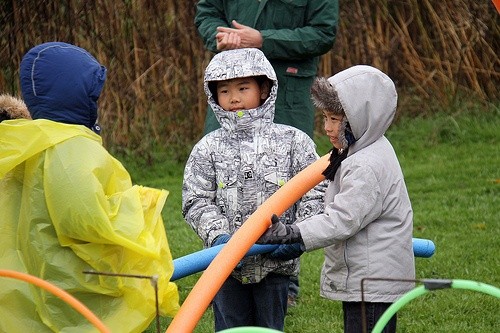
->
[182,48,330,333]
[1,42,180,333]
[255,65,416,333]
[194,0,338,306]
